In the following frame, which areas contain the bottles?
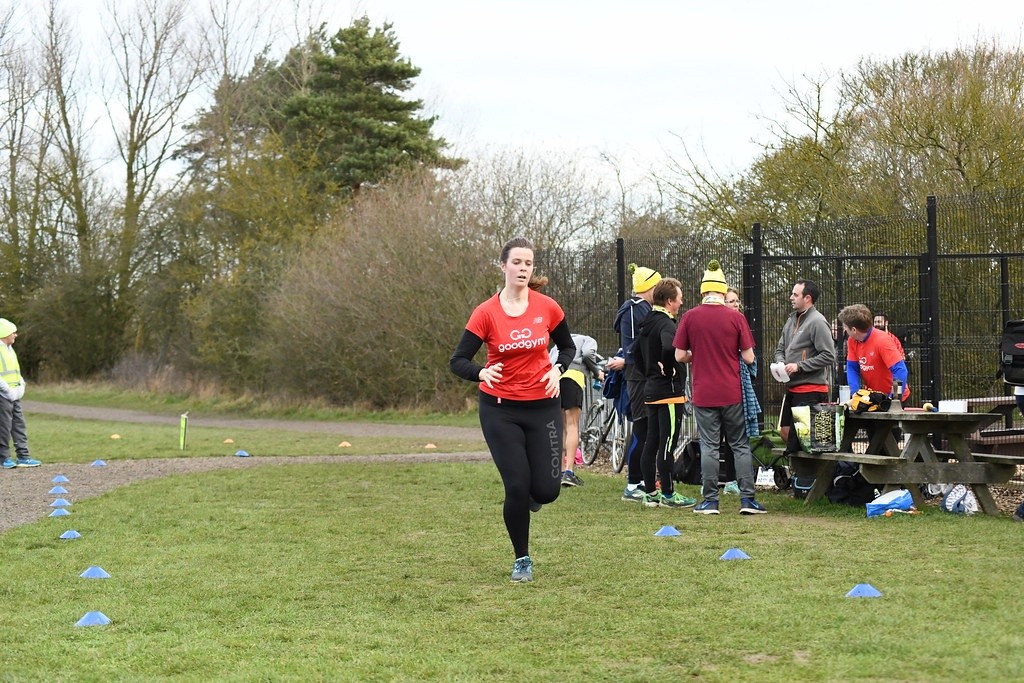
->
[593,363,603,390]
[770,362,790,383]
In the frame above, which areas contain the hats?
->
[628,263,662,293]
[700,260,727,294]
[0,318,17,339]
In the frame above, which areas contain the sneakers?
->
[693,497,720,515]
[621,484,645,504]
[528,495,542,512]
[739,496,767,515]
[643,492,662,508]
[637,483,647,492]
[0,456,17,469]
[509,557,533,584]
[15,457,41,468]
[560,470,584,488]
[659,492,697,508]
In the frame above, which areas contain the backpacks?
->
[998,319,1024,387]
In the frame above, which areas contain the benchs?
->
[935,451,1024,464]
[966,428,1024,445]
[771,448,906,466]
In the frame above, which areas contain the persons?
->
[772,280,835,449]
[838,304,913,455]
[0,318,43,468]
[449,237,578,582]
[548,332,605,487]
[671,259,769,515]
[1012,387,1024,521]
[603,263,698,509]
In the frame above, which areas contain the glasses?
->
[724,299,741,305]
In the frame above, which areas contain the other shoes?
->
[574,450,582,465]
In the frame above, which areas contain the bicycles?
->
[580,348,631,473]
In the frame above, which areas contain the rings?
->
[789,370,791,372]
[553,387,558,391]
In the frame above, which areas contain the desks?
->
[789,411,1016,516]
[938,396,1024,456]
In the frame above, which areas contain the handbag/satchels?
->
[671,441,736,485]
[791,398,845,453]
[825,461,873,508]
[865,490,917,519]
[792,473,817,500]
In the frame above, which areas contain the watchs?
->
[556,364,565,374]
